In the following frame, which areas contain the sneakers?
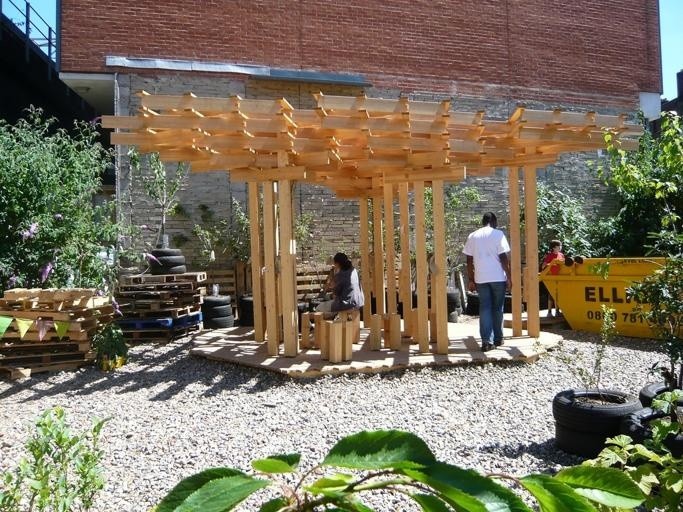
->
[546,312,563,319]
[481,338,503,352]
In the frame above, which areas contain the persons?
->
[316,253,365,319]
[542,240,564,318]
[461,212,512,351]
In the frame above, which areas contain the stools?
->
[300,307,432,364]
